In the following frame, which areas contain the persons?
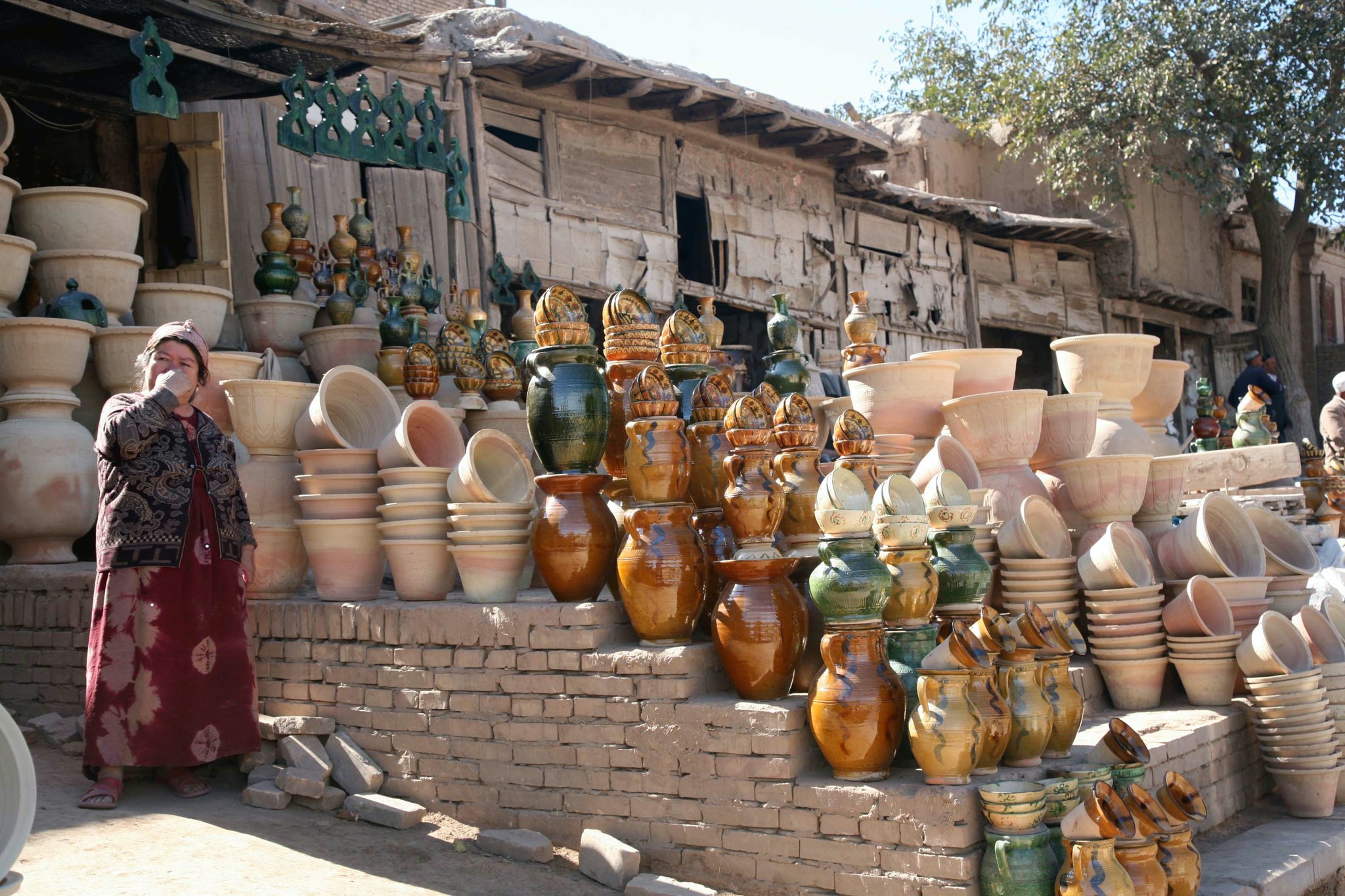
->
[1319,371,1345,466]
[1226,349,1290,444]
[77,317,263,810]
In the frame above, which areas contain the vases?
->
[0,94,1345,896]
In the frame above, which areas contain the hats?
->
[1262,353,1271,361]
[1331,371,1345,393]
[1244,349,1260,361]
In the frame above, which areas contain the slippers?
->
[78,778,126,808]
[164,765,210,798]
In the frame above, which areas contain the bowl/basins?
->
[921,469,978,528]
[871,473,928,546]
[1047,764,1114,802]
[724,396,772,450]
[534,284,712,364]
[750,382,780,415]
[977,781,1048,830]
[1035,777,1080,818]
[629,364,679,417]
[1108,762,1144,799]
[772,392,819,449]
[831,408,875,455]
[814,467,875,538]
[402,321,523,410]
[691,373,735,421]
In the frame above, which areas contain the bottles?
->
[666,364,719,426]
[878,546,940,768]
[697,291,887,396]
[981,823,1202,896]
[925,528,993,646]
[711,451,824,701]
[253,186,420,386]
[808,538,906,782]
[614,418,743,648]
[906,658,1084,784]
[524,348,664,603]
